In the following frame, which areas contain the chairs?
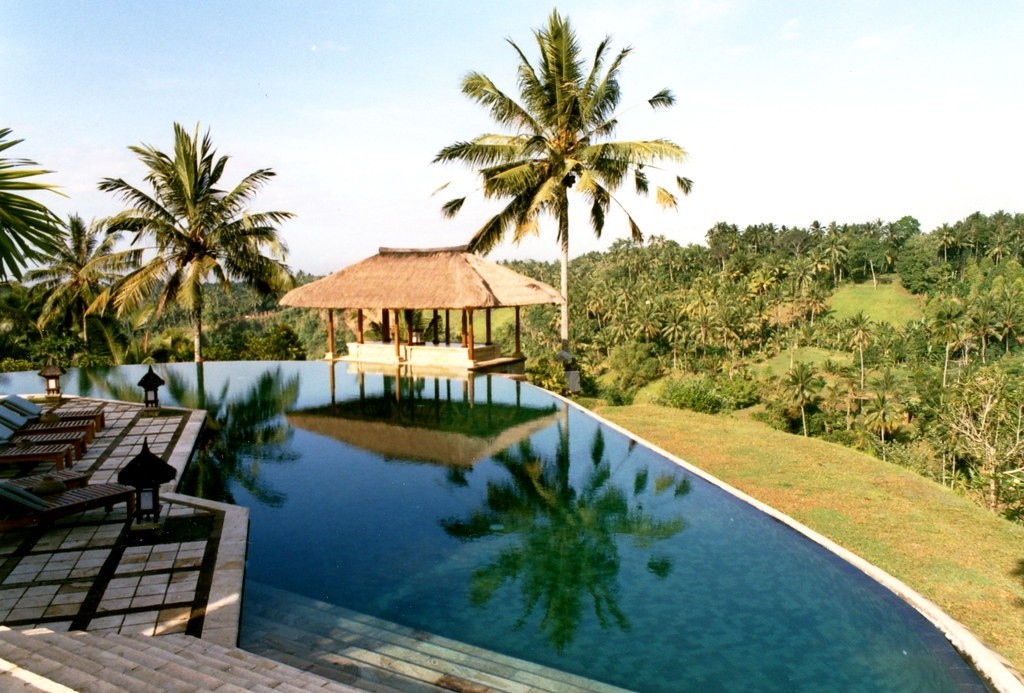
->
[0,394,105,432]
[0,405,96,443]
[0,423,88,459]
[0,481,138,533]
[0,469,89,492]
[0,443,74,469]
